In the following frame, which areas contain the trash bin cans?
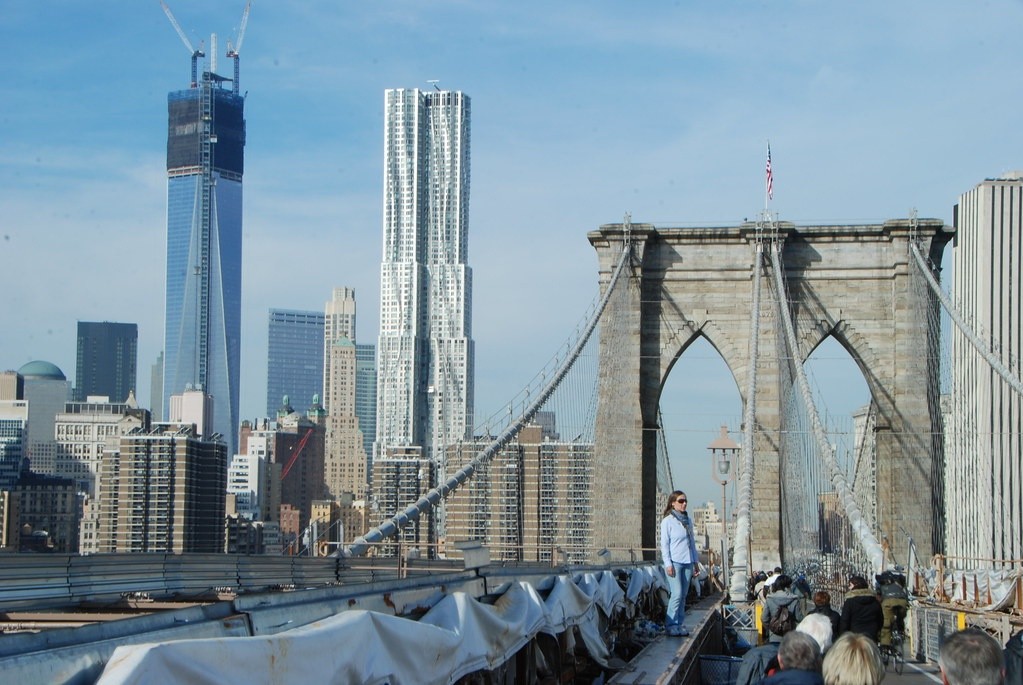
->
[701,654,743,685]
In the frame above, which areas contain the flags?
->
[766,147,774,199]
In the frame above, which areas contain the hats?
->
[759,573,766,579]
[775,567,781,572]
[798,576,805,581]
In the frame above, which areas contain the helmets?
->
[674,499,686,503]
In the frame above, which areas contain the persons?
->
[660,490,700,637]
[938,627,1023,685]
[747,567,812,606]
[736,574,885,685]
[875,570,911,664]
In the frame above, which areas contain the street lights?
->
[709,426,743,593]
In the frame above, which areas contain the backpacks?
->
[768,606,797,636]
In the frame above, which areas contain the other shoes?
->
[679,625,689,636]
[665,625,681,636]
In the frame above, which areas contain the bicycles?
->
[878,607,906,675]
